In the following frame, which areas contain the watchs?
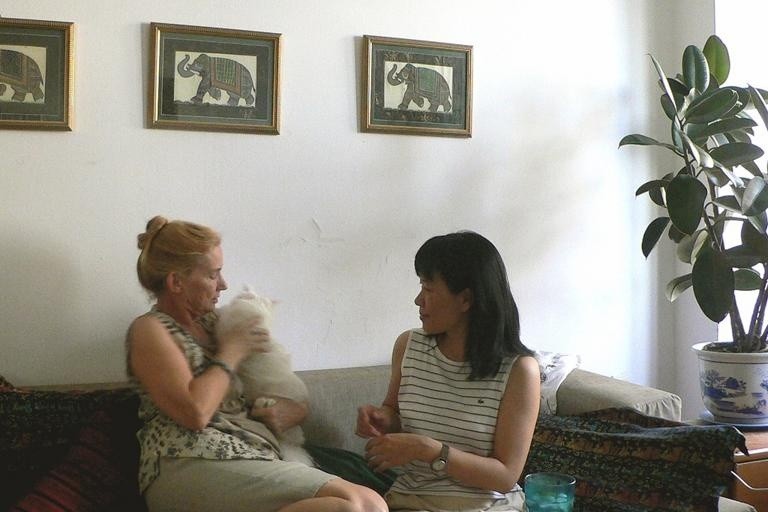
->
[430,442,450,476]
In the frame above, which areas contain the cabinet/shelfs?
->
[685,414,768,511]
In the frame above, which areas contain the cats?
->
[210,283,323,467]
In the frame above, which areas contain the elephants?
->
[387,61,453,115]
[0,48,45,103]
[176,51,258,108]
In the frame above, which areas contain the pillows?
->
[516,405,747,510]
[0,375,139,510]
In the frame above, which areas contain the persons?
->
[352,227,546,512]
[123,214,395,512]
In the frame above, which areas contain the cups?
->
[525,471,576,512]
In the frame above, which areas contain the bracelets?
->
[206,360,237,377]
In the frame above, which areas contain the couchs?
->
[2,363,754,511]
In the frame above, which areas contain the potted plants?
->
[620,31,768,430]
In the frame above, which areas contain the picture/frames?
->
[0,18,75,131]
[147,22,283,133]
[360,33,473,137]
